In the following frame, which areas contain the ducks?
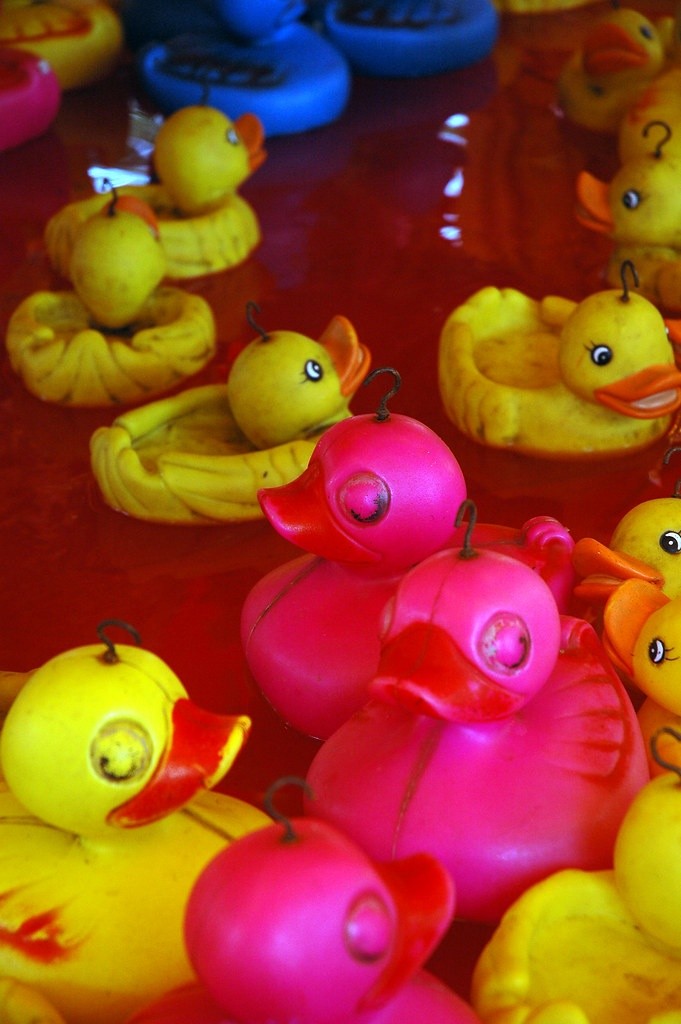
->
[1,1,681,1024]
